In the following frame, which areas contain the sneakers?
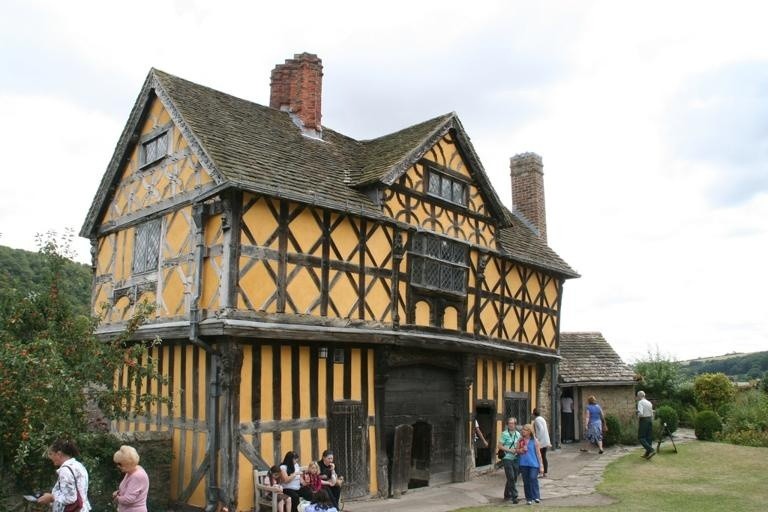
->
[537,470,551,480]
[525,497,532,507]
[535,496,542,506]
[562,438,575,445]
[580,445,589,453]
[502,492,521,504]
[644,448,659,459]
[595,446,605,455]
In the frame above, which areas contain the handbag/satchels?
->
[498,445,509,459]
[58,488,84,512]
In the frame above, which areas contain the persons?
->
[499,417,521,504]
[476,419,488,466]
[637,390,656,460]
[532,408,551,478]
[36,437,92,512]
[516,424,545,505]
[112,444,149,512]
[580,395,608,453]
[265,450,339,512]
[561,394,573,443]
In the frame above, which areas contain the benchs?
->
[253,467,342,511]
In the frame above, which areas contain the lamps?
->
[318,342,328,360]
[507,357,514,372]
